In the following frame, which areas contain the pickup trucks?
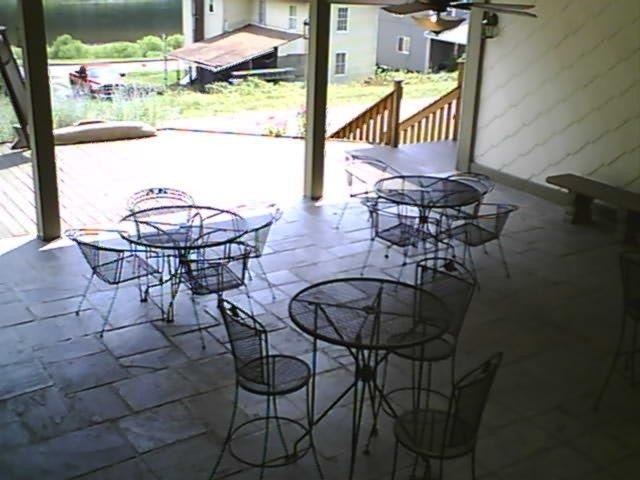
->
[68,61,129,102]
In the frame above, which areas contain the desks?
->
[288,277,452,479]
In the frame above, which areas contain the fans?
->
[379,1,541,21]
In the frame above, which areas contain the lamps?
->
[410,6,466,39]
[482,11,501,41]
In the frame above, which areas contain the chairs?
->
[593,239,640,419]
[66,187,282,350]
[363,257,502,478]
[209,298,325,479]
[333,157,519,294]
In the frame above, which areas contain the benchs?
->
[544,172,638,248]
